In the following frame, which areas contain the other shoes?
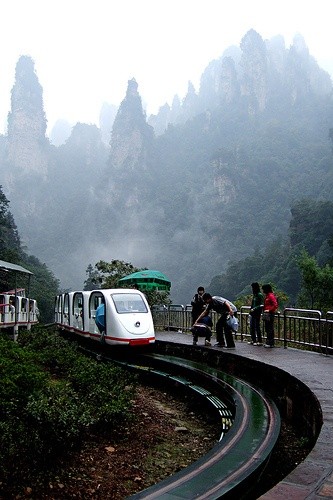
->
[247,340,274,348]
[214,342,235,350]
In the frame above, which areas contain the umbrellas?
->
[117,270,171,291]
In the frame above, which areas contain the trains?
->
[2,287,26,298]
[53,287,157,347]
[0,294,41,329]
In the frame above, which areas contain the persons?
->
[95,298,105,341]
[247,282,264,346]
[260,284,278,348]
[9,304,15,314]
[192,293,238,349]
[191,287,206,326]
[191,310,213,346]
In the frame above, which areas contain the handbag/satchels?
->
[227,315,238,331]
[262,312,271,321]
